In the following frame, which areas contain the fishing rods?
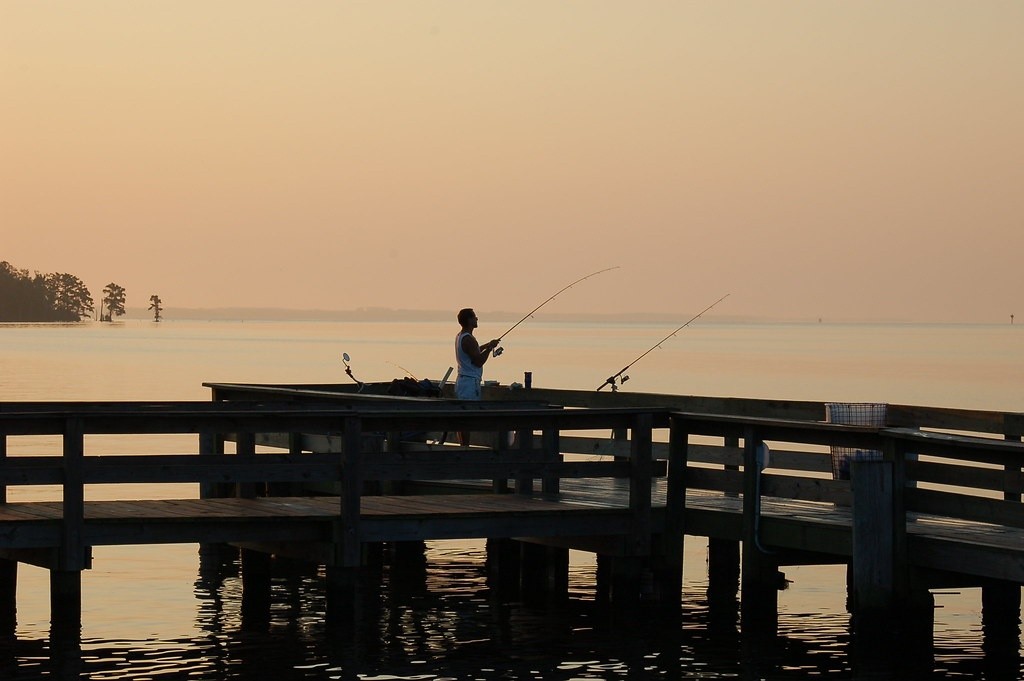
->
[490,266,621,358]
[595,292,733,392]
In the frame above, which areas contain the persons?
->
[454,309,499,449]
